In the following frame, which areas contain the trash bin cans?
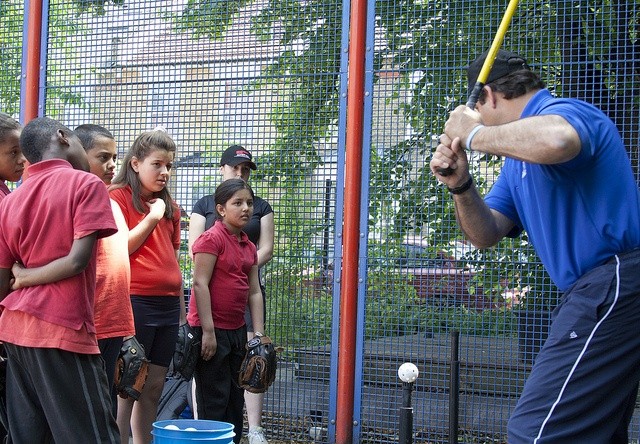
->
[512,310,552,364]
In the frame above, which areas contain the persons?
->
[71,124,136,395]
[0,116,122,443]
[108,127,181,443]
[429,49,640,444]
[0,112,27,444]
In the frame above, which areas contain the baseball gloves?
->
[237,334,277,393]
[173,323,203,382]
[114,335,151,401]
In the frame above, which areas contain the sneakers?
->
[247,425,269,443]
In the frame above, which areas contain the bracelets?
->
[465,125,485,150]
[449,176,472,194]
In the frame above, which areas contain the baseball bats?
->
[435,0,520,177]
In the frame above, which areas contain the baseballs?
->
[398,362,419,383]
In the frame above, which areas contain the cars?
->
[304,236,505,307]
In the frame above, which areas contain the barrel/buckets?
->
[150,419,236,444]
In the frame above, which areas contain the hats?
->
[467,48,526,95]
[220,145,257,171]
